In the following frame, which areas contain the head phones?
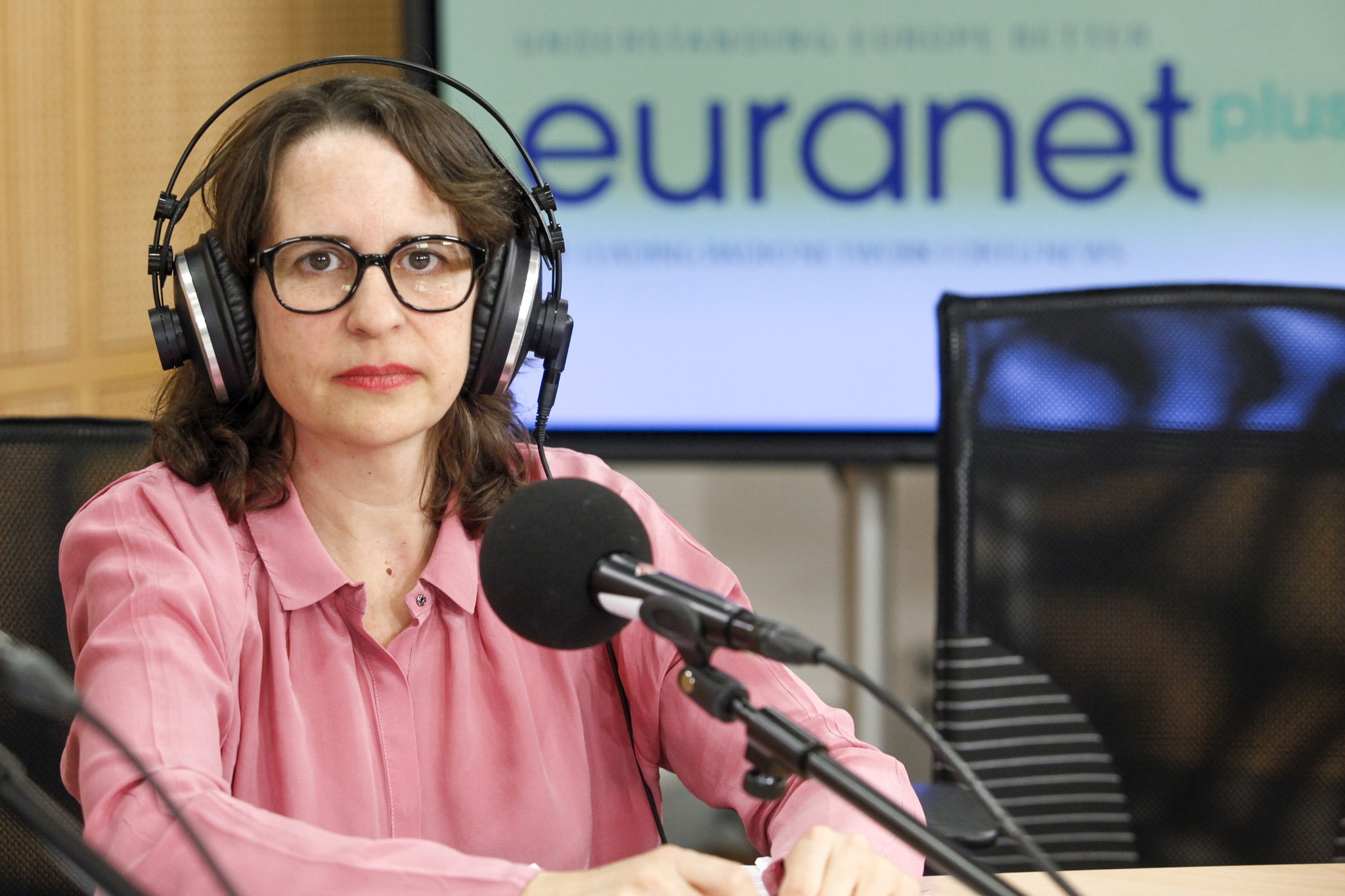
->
[150,60,567,408]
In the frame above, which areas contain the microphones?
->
[480,480,823,668]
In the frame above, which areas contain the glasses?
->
[246,234,487,315]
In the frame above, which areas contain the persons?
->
[55,78,928,896]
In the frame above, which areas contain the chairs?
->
[0,408,169,896]
[926,275,1345,865]
